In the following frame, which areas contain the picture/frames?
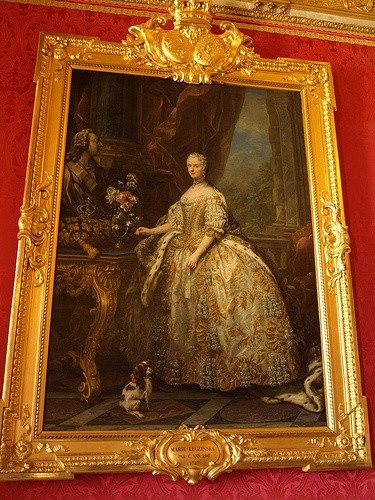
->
[0,0,375,483]
